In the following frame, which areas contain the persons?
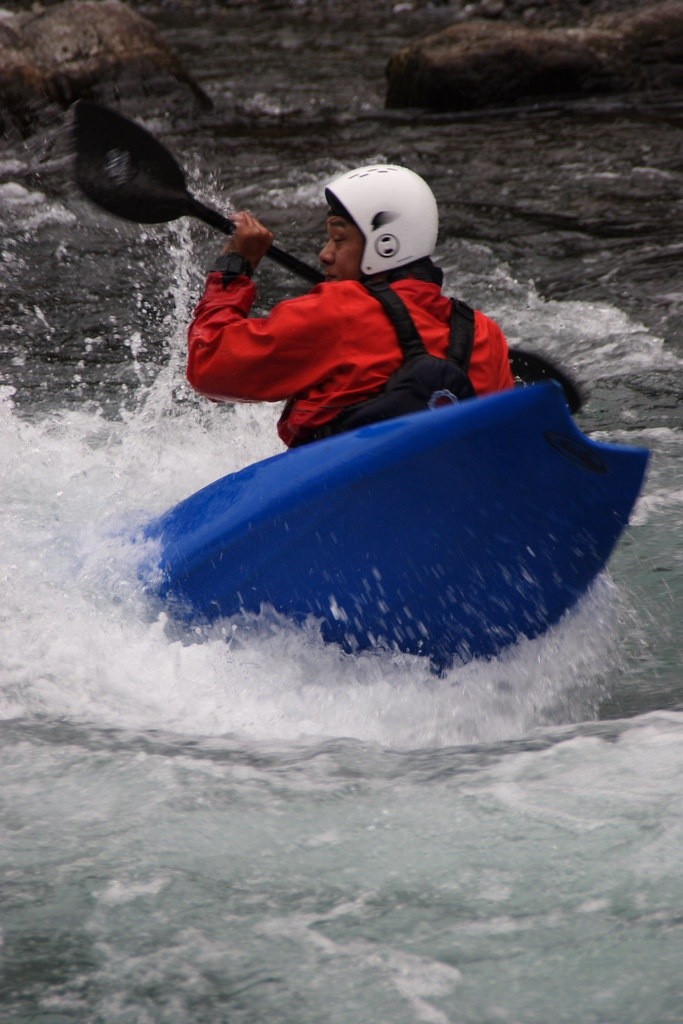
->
[186,164,517,446]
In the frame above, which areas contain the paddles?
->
[70,91,587,420]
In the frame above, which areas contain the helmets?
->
[325,164,440,276]
